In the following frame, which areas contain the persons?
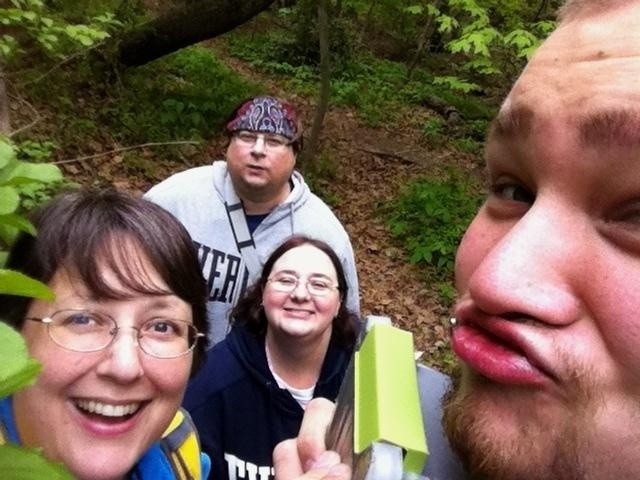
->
[1,183,213,480]
[255,0,639,479]
[173,233,368,478]
[140,92,362,349]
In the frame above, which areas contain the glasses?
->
[24,308,205,358]
[268,275,338,296]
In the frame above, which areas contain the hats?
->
[224,96,302,145]
